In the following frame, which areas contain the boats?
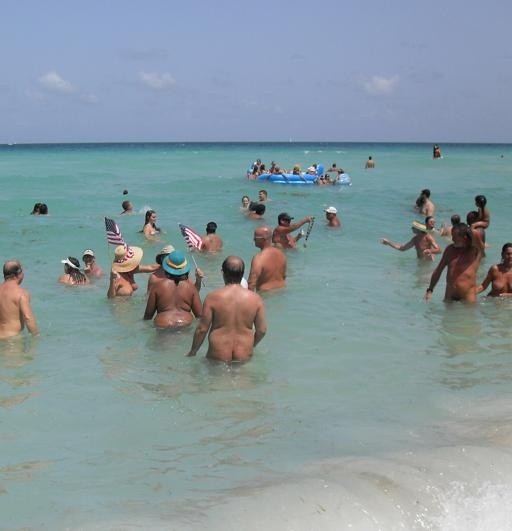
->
[255,163,324,184]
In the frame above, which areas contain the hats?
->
[410,220,427,232]
[112,245,143,272]
[61,258,78,269]
[156,245,190,275]
[278,213,294,220]
[323,207,337,213]
[83,250,93,257]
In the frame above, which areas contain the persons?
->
[0,144,512,363]
